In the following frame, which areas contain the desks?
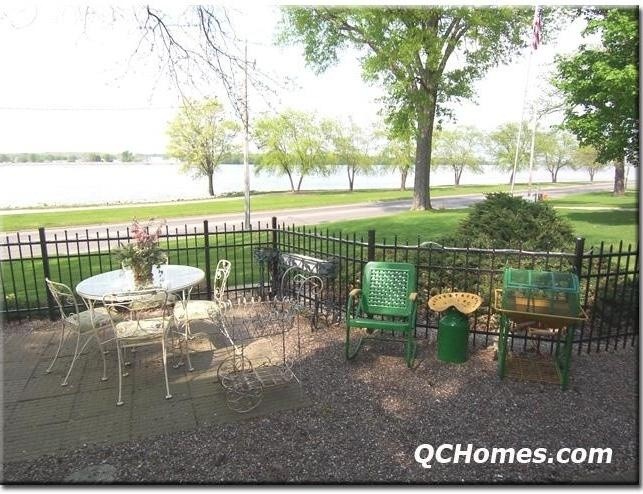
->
[75,265,206,381]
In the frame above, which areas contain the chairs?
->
[173,258,242,369]
[121,255,170,352]
[103,289,172,406]
[45,277,119,387]
[345,261,419,369]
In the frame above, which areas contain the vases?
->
[132,259,154,280]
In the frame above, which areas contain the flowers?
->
[115,215,169,277]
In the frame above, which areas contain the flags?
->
[530,6,545,50]
